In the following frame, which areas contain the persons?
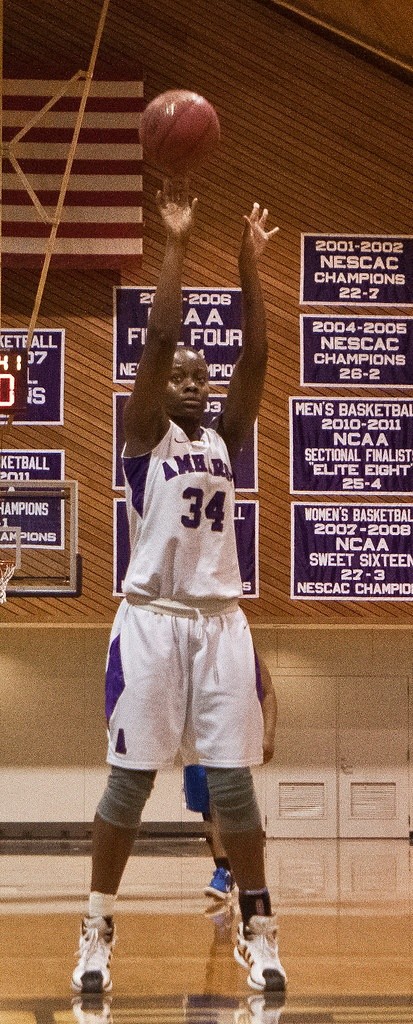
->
[69,166,287,993]
[183,644,278,901]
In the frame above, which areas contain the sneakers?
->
[235,994,288,1024]
[234,913,288,992]
[70,914,115,991]
[203,902,235,930]
[205,868,236,899]
[71,996,113,1024]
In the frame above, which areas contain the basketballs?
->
[137,87,222,174]
